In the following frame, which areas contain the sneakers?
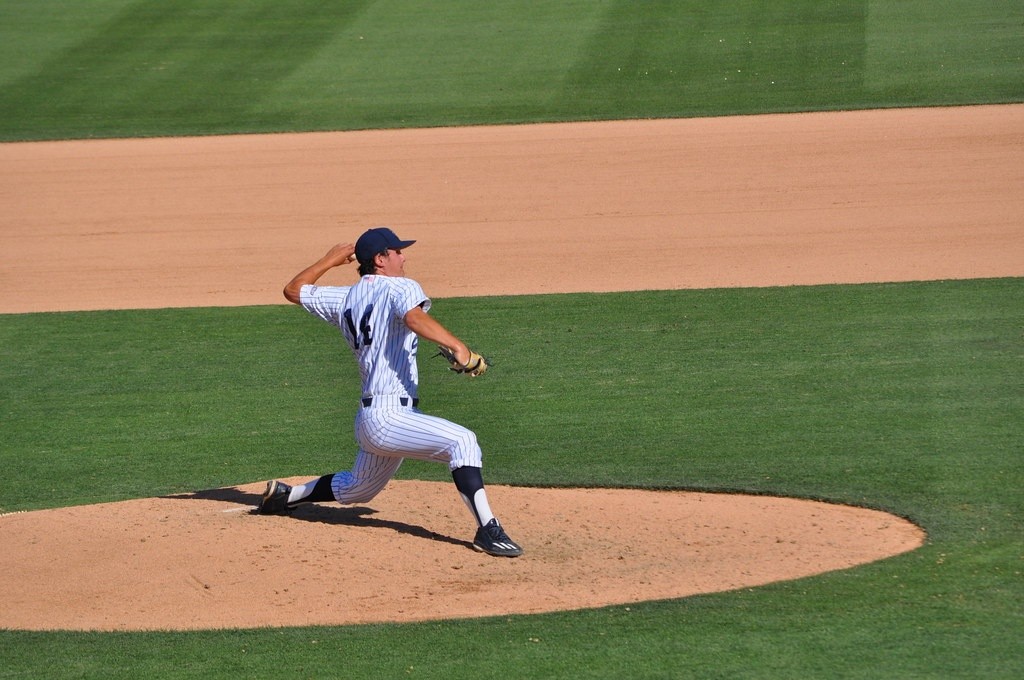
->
[473,518,523,557]
[257,481,298,515]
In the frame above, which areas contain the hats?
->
[355,227,416,263]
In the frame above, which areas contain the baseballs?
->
[348,253,357,261]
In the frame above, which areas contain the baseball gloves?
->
[429,344,496,378]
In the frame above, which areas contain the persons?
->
[257,227,522,557]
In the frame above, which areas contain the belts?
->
[362,398,419,407]
